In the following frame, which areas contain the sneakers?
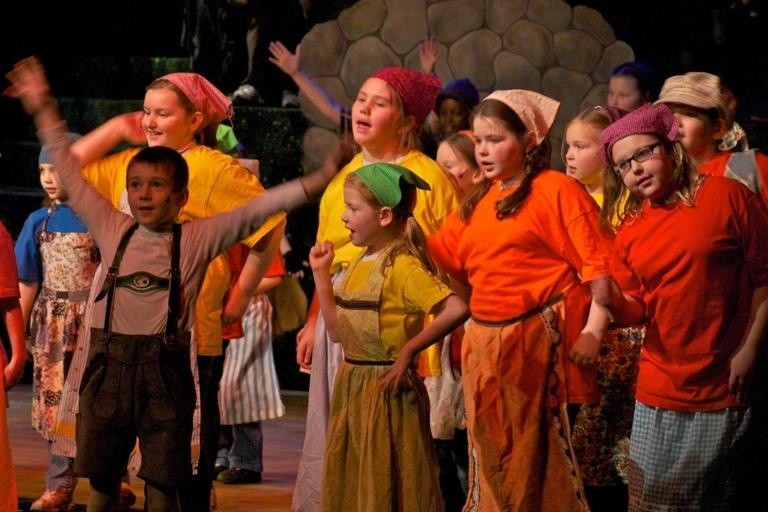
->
[215,465,262,484]
[29,489,73,512]
[119,478,137,509]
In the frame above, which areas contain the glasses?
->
[613,139,664,177]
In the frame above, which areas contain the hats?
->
[652,71,738,131]
